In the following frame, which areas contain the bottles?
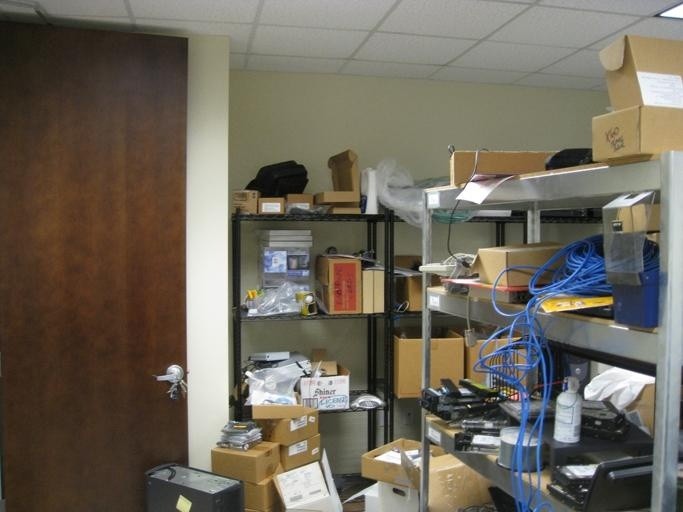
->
[552,388,583,442]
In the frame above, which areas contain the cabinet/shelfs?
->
[233,210,392,460]
[424,153,680,509]
[372,208,601,454]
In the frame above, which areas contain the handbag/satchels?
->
[245,160,309,197]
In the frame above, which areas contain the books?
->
[217,420,263,451]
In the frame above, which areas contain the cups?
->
[496,425,544,471]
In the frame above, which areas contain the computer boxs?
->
[143,463,244,511]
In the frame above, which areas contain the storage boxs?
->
[606,265,661,331]
[394,253,437,313]
[384,323,464,402]
[231,148,386,316]
[466,335,529,389]
[209,402,489,512]
[448,149,557,189]
[472,240,571,286]
[620,382,655,441]
[296,362,352,411]
[587,34,682,162]
